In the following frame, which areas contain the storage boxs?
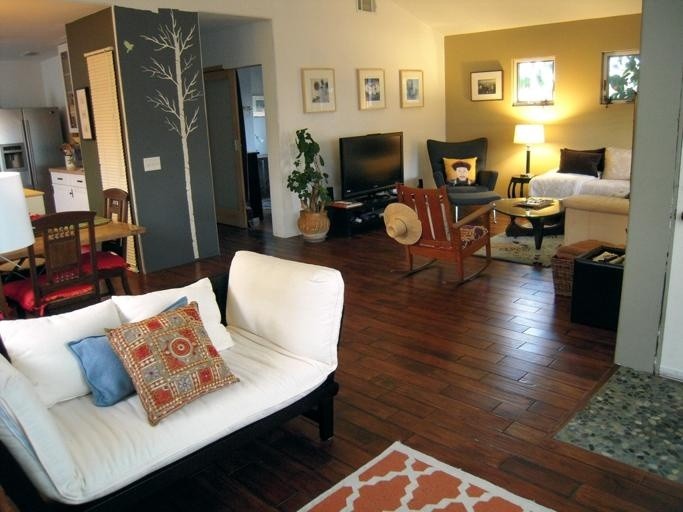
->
[569,245,626,330]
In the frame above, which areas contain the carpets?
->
[552,363,683,486]
[294,442,554,510]
[472,220,566,267]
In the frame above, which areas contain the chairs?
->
[78,191,133,297]
[426,137,498,223]
[396,182,496,284]
[3,209,102,316]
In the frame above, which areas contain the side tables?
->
[507,175,536,198]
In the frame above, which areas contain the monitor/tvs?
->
[339,132,404,202]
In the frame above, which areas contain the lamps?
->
[0,171,35,259]
[513,124,544,177]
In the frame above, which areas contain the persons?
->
[447,161,476,186]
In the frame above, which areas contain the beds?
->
[527,145,631,200]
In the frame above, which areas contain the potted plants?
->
[285,128,331,243]
[58,143,76,170]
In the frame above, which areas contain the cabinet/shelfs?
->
[47,166,90,212]
[55,39,80,133]
[317,193,398,239]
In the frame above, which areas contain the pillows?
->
[556,148,602,178]
[1,300,122,406]
[441,157,477,188]
[104,298,233,427]
[564,147,606,174]
[603,146,631,180]
[65,297,193,405]
[119,276,235,362]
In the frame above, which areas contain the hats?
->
[383,203,423,246]
[452,160,471,171]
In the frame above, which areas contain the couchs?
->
[0,250,347,512]
[562,195,630,247]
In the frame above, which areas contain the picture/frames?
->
[250,94,265,118]
[73,85,96,142]
[470,69,504,101]
[356,68,387,110]
[400,70,426,110]
[299,66,337,115]
